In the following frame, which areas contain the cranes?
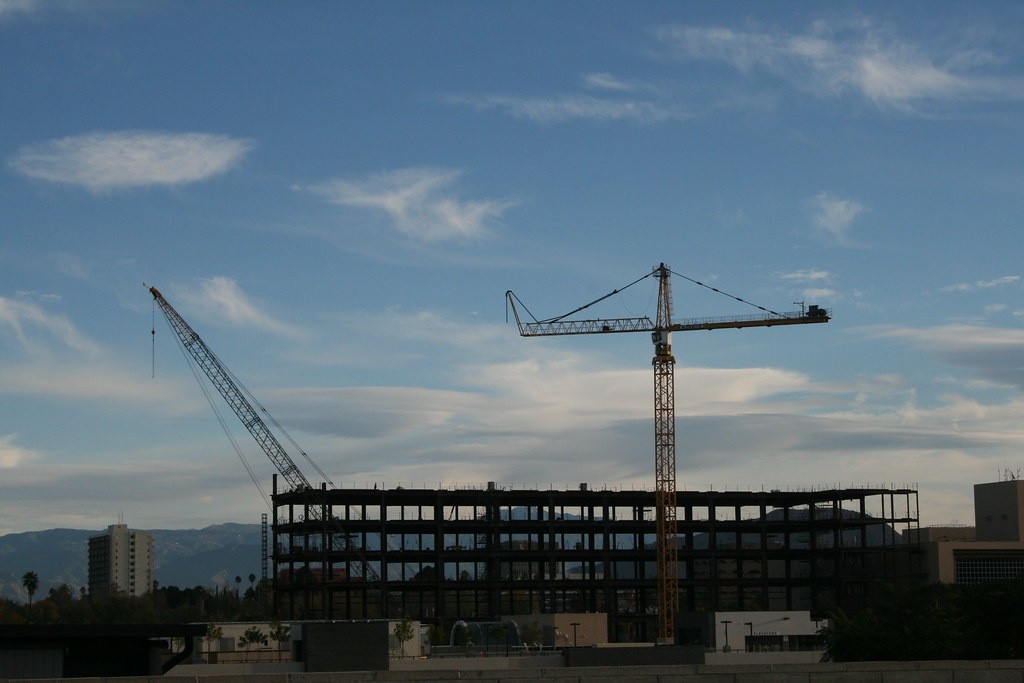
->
[501,261,835,641]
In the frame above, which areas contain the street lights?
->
[720,621,732,646]
[744,622,753,652]
[486,624,494,651]
[570,623,580,647]
[504,626,508,657]
[552,627,558,650]
